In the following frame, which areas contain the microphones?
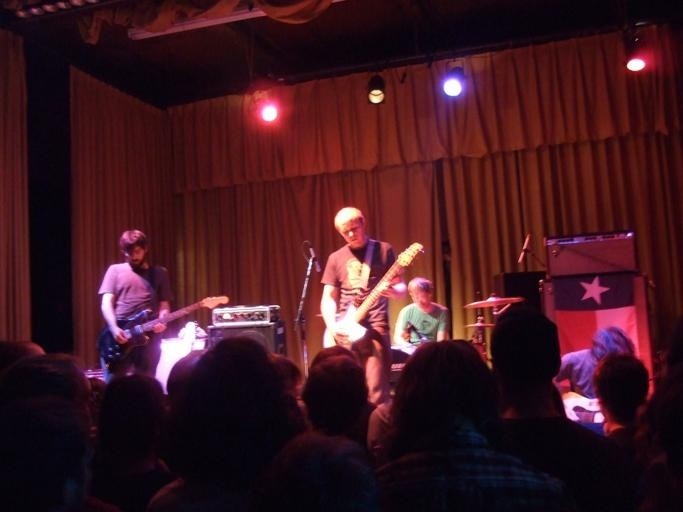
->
[406,319,429,343]
[516,231,532,264]
[300,237,322,272]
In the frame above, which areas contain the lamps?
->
[622,30,647,72]
[443,58,464,97]
[367,74,385,105]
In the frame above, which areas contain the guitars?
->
[97,296,229,362]
[323,243,424,348]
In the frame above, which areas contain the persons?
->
[394,277,450,353]
[97,230,172,380]
[1,304,682,510]
[319,208,414,407]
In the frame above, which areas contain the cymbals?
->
[465,298,525,309]
[466,324,494,327]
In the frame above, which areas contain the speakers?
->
[495,270,546,321]
[207,320,288,356]
